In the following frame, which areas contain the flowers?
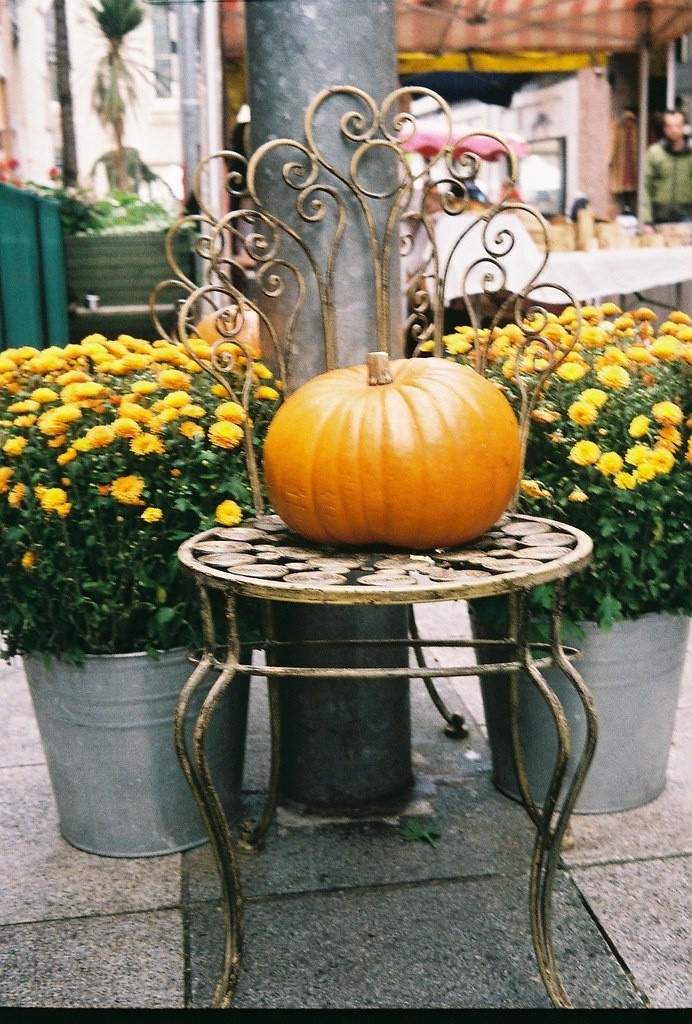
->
[422,303,692,618]
[0,335,284,656]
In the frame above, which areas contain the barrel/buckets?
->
[14,642,238,858]
[462,602,688,817]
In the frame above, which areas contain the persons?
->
[643,109,691,233]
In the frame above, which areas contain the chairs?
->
[155,85,607,1009]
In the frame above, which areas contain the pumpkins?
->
[261,352,522,547]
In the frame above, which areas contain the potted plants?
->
[66,0,198,342]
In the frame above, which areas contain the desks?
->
[410,191,692,315]
